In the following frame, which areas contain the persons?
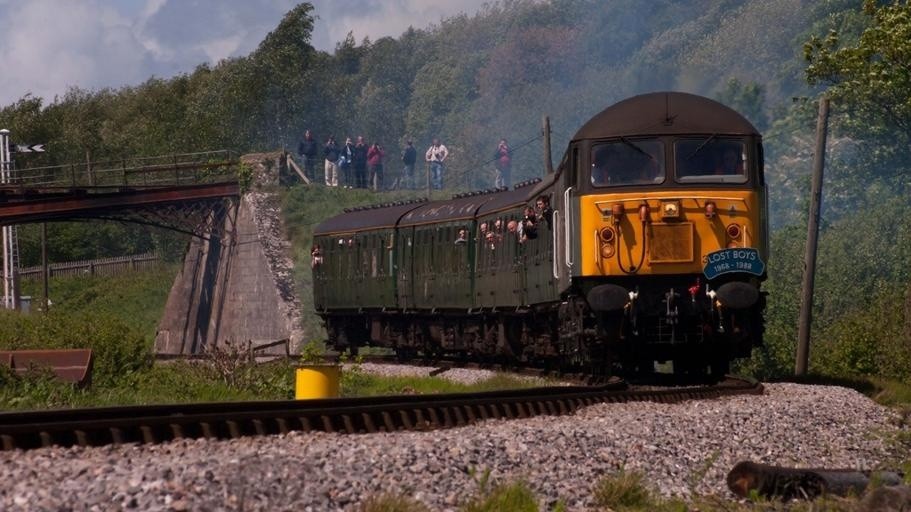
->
[311,244,324,269]
[426,138,449,190]
[590,148,633,185]
[454,195,554,245]
[324,134,385,190]
[713,148,743,175]
[401,139,416,189]
[298,129,317,182]
[495,138,511,191]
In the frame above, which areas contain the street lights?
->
[0,129,10,310]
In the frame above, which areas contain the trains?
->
[312,92,769,375]
